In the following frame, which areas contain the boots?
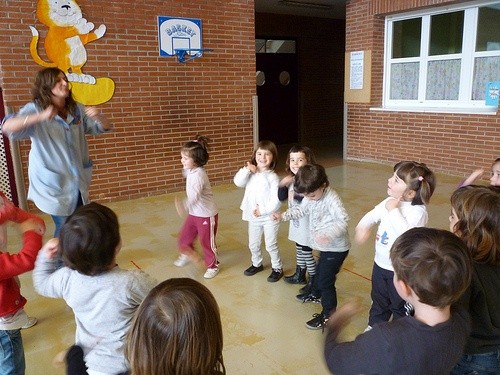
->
[299,274,315,292]
[283,265,308,284]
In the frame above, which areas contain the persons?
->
[32,201,157,374]
[459,157,500,187]
[0,189,46,329]
[448,185,500,375]
[271,164,352,330]
[0,219,42,375]
[174,134,220,278]
[322,226,473,375]
[233,142,284,283]
[1,66,113,239]
[55,277,227,375]
[277,144,318,293]
[354,161,437,333]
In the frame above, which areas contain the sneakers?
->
[296,292,321,304]
[305,310,329,334]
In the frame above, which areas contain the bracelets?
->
[24,115,29,128]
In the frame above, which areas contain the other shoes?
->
[267,268,284,282]
[203,267,220,278]
[174,253,193,266]
[21,317,38,329]
[362,325,372,334]
[244,264,264,275]
